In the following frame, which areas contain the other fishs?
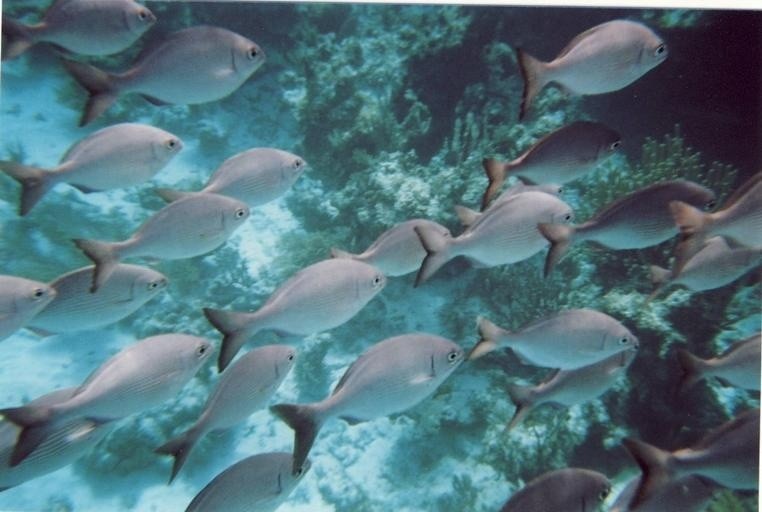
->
[2,121,759,512]
